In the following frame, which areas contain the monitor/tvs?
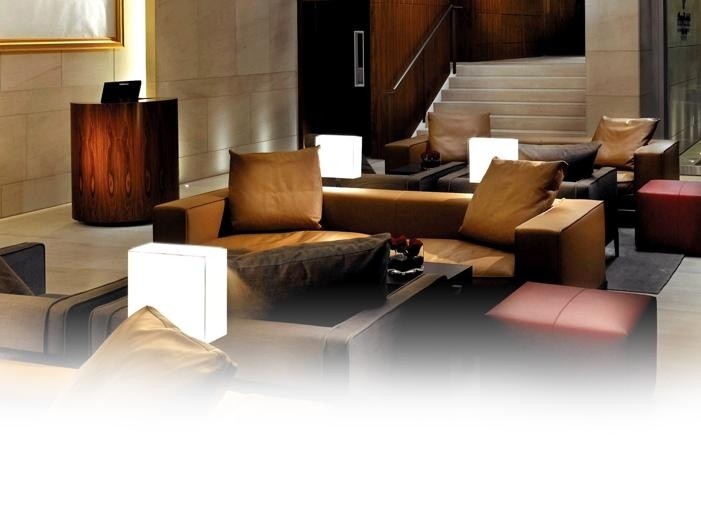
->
[101,80,141,103]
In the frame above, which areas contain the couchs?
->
[150,131,678,293]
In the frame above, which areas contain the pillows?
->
[428,111,492,161]
[588,115,662,171]
[232,233,389,327]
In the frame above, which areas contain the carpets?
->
[606,227,685,295]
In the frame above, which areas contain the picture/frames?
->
[2,0,126,53]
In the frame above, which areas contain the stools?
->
[639,180,701,258]
[483,282,658,393]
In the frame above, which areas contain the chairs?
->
[1,240,453,381]
